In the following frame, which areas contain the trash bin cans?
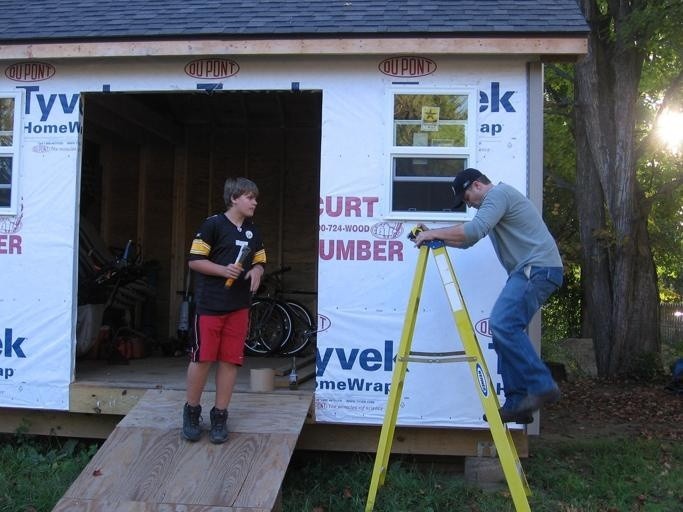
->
[249,367,275,393]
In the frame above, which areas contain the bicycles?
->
[244,272,312,358]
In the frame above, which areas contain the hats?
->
[450,168,482,209]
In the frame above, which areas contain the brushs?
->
[289,357,298,385]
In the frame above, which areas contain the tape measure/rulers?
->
[409,227,424,239]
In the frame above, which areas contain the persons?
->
[179,176,268,444]
[410,167,565,426]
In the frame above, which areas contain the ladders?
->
[365,239,533,511]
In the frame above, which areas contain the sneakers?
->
[210,406,228,443]
[182,401,202,441]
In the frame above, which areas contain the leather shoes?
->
[483,386,561,423]
